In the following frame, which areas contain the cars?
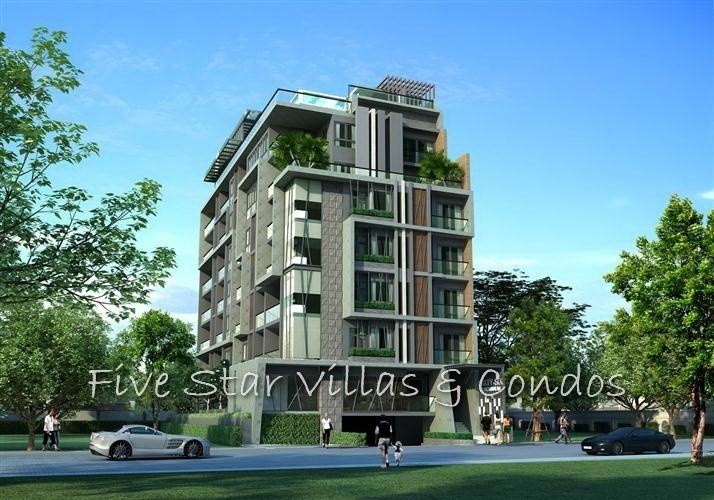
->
[581,426,676,455]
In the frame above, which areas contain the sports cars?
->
[88,423,211,462]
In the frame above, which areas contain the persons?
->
[554,413,575,445]
[40,408,61,452]
[374,413,393,468]
[493,413,502,446]
[502,414,511,445]
[48,413,60,448]
[321,412,333,449]
[480,411,493,445]
[391,440,404,467]
